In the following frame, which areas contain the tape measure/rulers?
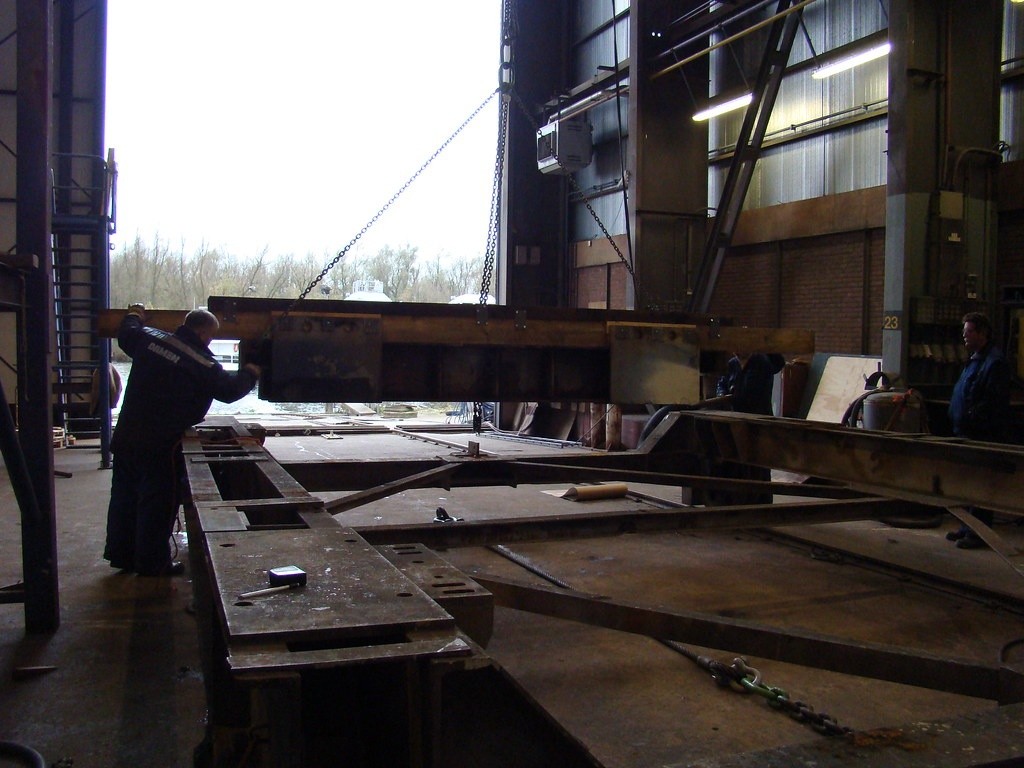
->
[268,564,309,588]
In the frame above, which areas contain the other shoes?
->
[110,560,185,577]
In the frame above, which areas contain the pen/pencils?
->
[239,582,300,599]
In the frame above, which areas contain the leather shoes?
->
[945,530,989,549]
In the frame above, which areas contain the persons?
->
[944,313,1010,549]
[718,351,775,503]
[101,302,262,579]
